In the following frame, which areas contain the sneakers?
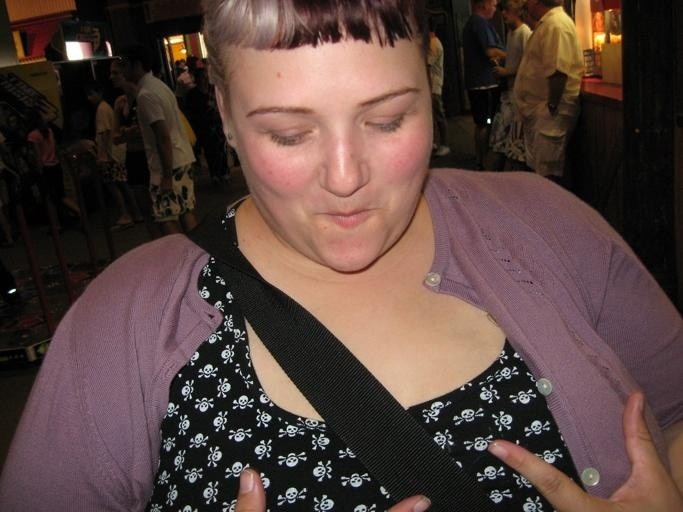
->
[435,145,450,156]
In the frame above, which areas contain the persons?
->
[0,0,681,509]
[423,1,584,180]
[1,39,232,236]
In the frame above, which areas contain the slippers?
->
[110,216,143,233]
